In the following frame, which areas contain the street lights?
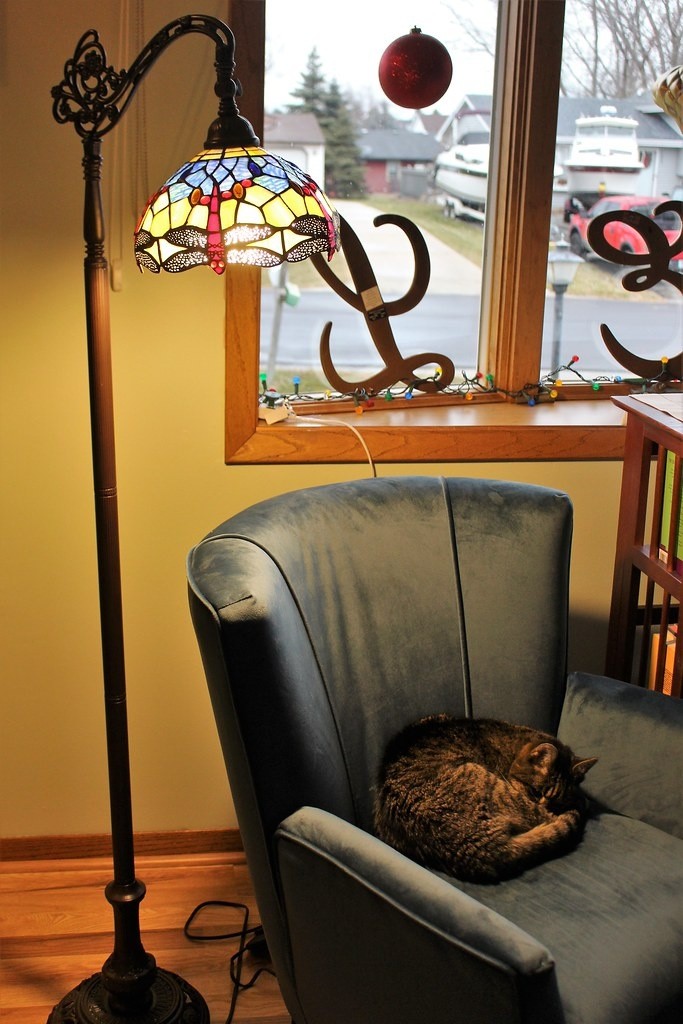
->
[547,233,587,381]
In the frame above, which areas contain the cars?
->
[568,195,683,264]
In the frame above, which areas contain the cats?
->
[374,713,599,885]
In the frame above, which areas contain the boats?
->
[564,103,647,209]
[434,108,567,215]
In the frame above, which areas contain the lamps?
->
[50,13,339,1024]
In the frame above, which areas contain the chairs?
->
[187,476,683,1023]
